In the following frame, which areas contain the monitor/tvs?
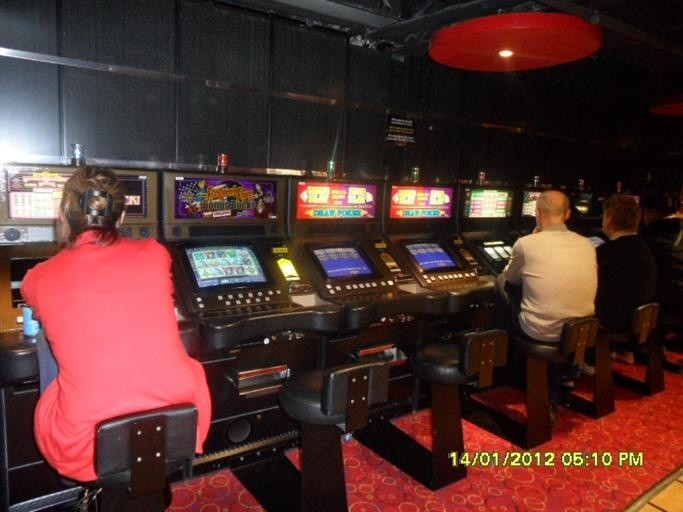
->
[474,234,516,267]
[584,235,607,251]
[397,236,465,274]
[304,239,383,285]
[10,258,63,308]
[179,241,275,293]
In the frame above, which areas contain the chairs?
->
[350,327,514,490]
[74,398,200,511]
[506,299,662,421]
[228,352,395,511]
[458,314,601,453]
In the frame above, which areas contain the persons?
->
[18,165,213,485]
[494,189,683,392]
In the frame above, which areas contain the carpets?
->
[165,346,683,512]
